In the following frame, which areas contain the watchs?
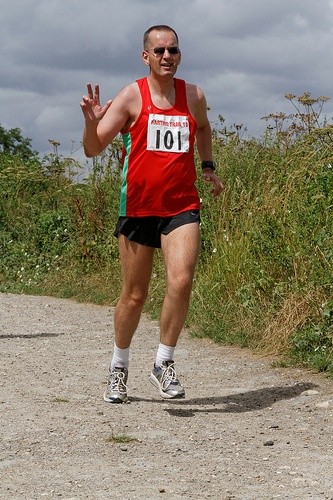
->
[201,160,216,171]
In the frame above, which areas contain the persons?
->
[80,25,224,403]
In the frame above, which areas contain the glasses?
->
[145,47,181,54]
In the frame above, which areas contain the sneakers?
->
[104,362,128,402]
[149,360,185,399]
